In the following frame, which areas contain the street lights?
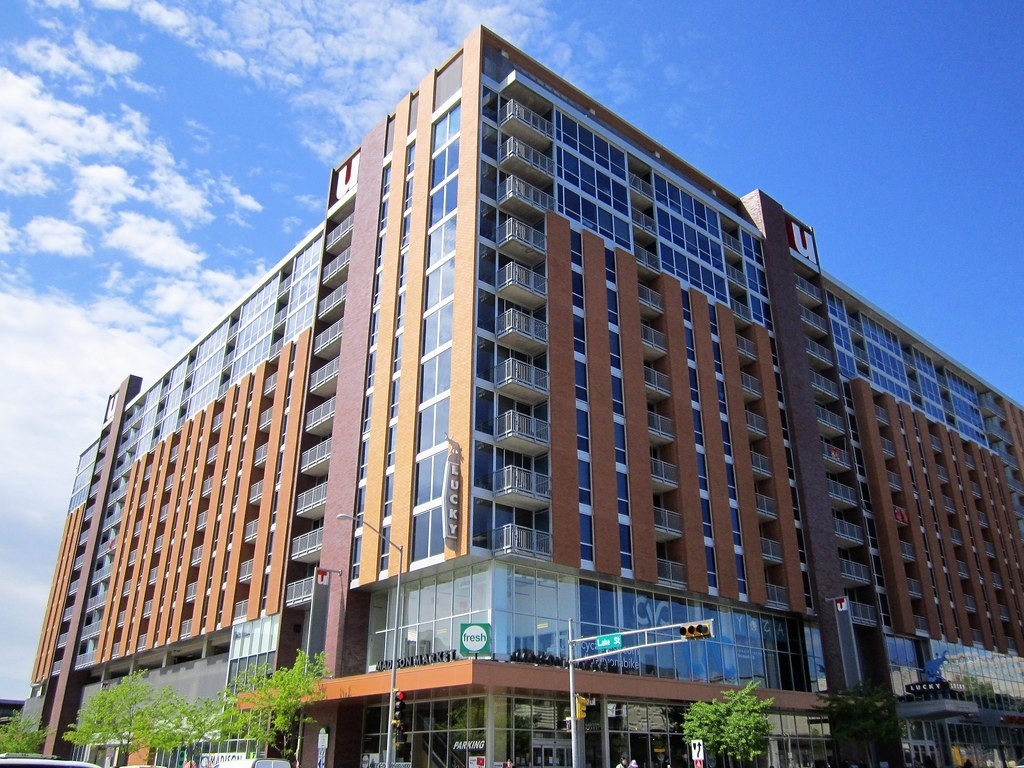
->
[336,514,403,768]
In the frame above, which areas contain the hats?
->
[630,760,638,768]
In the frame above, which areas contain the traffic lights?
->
[680,624,710,638]
[576,694,587,720]
[394,691,405,720]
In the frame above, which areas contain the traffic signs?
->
[594,633,622,649]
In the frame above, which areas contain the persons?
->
[616,753,637,768]
